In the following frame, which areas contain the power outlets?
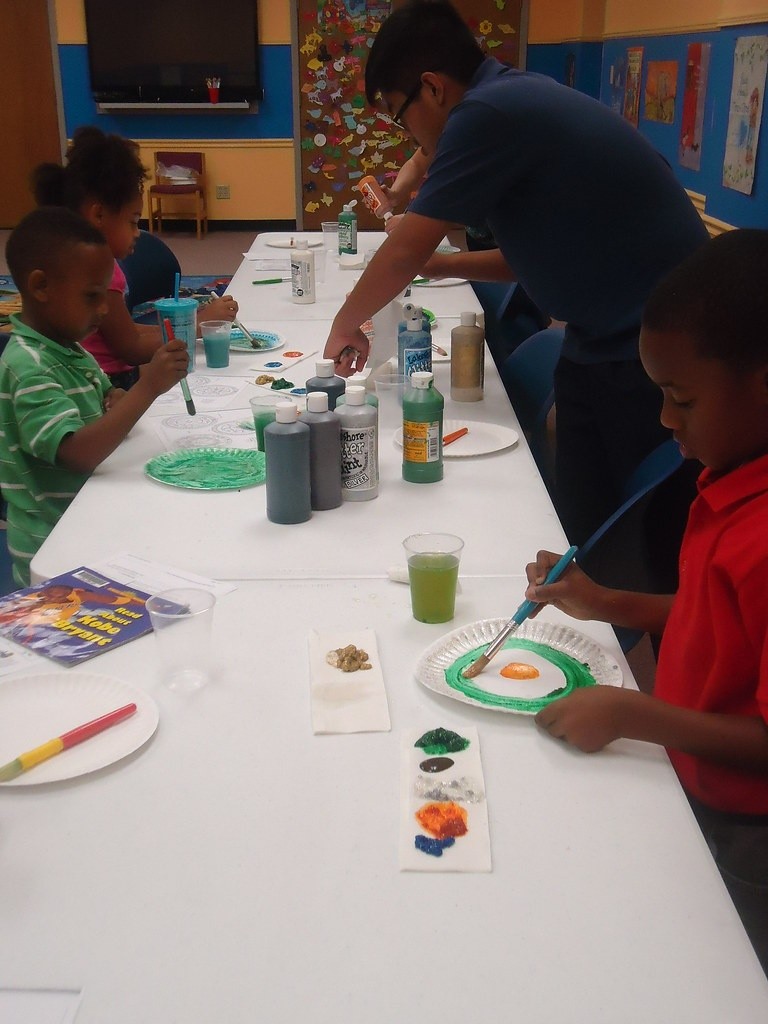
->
[216,185,230,199]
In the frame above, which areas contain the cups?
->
[403,531,465,624]
[154,297,199,373]
[248,395,292,454]
[144,586,217,695]
[374,374,411,429]
[321,221,339,256]
[199,319,232,368]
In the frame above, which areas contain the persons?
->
[523,229,768,964]
[0,206,190,584]
[32,126,238,391]
[323,2,717,661]
[363,143,518,282]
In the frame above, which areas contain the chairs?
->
[147,152,207,240]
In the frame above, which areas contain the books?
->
[0,563,191,668]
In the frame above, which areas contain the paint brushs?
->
[205,76,221,89]
[211,290,262,349]
[431,343,448,357]
[404,280,412,297]
[464,546,578,678]
[252,277,292,285]
[442,428,468,446]
[164,319,197,417]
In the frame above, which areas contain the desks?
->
[0,232,768,1024]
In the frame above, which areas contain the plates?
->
[394,419,520,458]
[201,329,285,353]
[0,671,160,789]
[413,274,468,287]
[411,617,625,717]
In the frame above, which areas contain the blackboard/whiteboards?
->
[83,0,265,104]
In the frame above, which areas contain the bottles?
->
[263,358,379,525]
[357,175,394,220]
[289,239,316,305]
[401,372,445,484]
[397,319,433,383]
[450,311,486,403]
[337,200,358,255]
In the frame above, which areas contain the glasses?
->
[390,63,445,130]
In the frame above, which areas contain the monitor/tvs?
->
[84,0,261,103]
